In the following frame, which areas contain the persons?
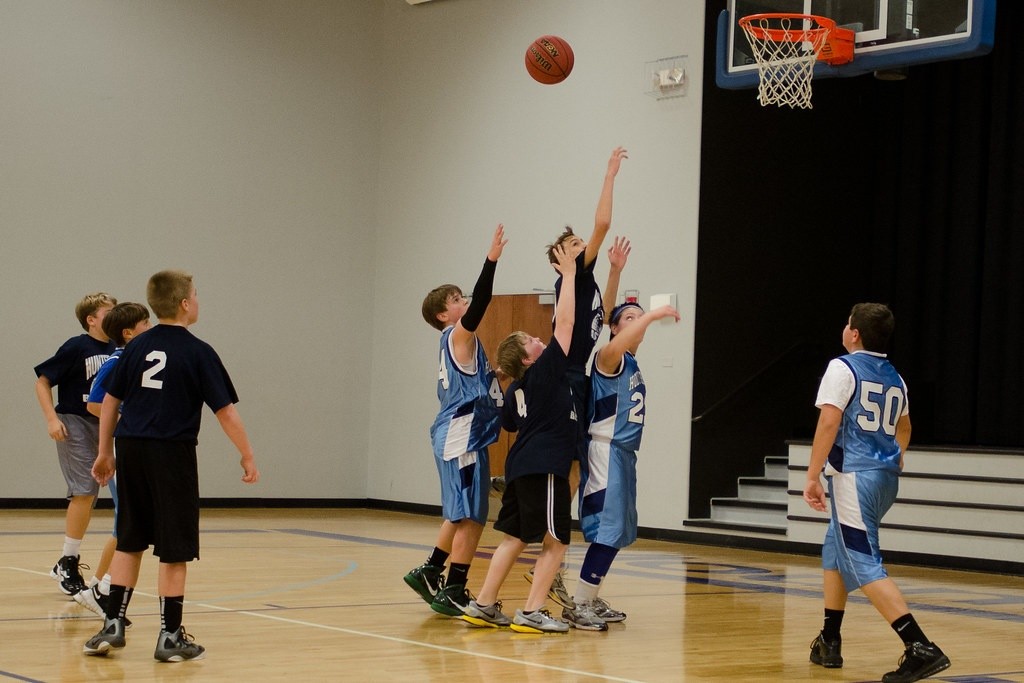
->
[803,302,952,683]
[34,294,119,596]
[561,302,680,631]
[73,301,153,630]
[462,241,578,633]
[489,144,632,609]
[404,224,508,616]
[84,270,261,661]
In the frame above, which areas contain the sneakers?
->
[881,641,951,683]
[50,554,89,595]
[524,565,576,610]
[562,600,627,631]
[83,617,126,656]
[154,625,206,662]
[462,600,513,630]
[810,629,844,668]
[509,605,569,634]
[403,565,477,618]
[73,584,133,630]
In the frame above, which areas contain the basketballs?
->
[524,35,574,86]
